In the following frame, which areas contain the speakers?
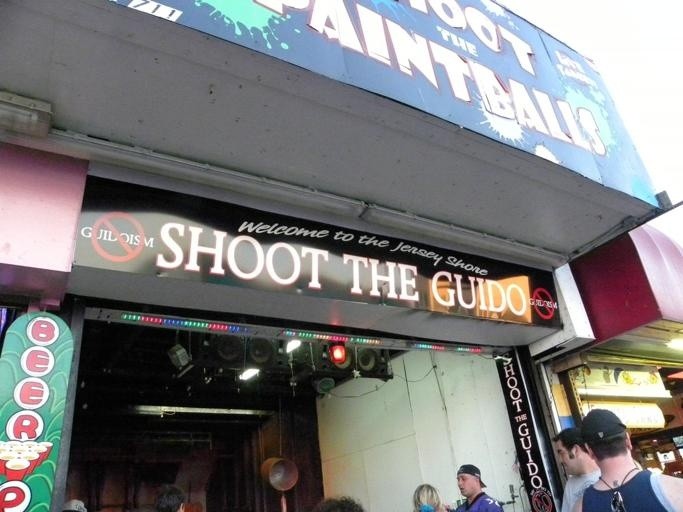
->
[199,330,394,379]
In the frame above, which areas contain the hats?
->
[457,464,487,488]
[580,408,626,444]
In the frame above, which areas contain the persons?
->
[152,483,187,512]
[571,408,683,511]
[412,483,445,512]
[551,427,602,512]
[444,464,504,512]
